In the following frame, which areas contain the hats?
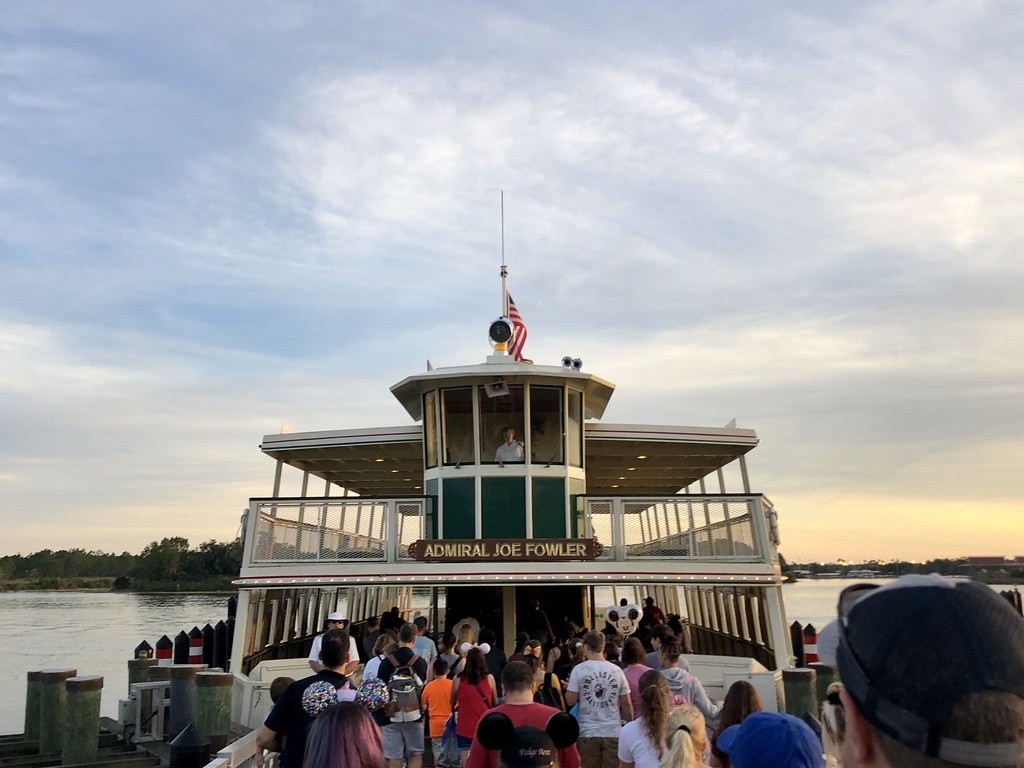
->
[514,633,528,642]
[476,713,580,767]
[643,597,654,600]
[717,710,823,767]
[327,612,346,620]
[816,571,1024,768]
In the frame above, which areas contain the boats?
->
[226,186,796,741]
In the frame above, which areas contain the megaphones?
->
[484,381,510,398]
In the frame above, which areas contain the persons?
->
[256,574,1024,768]
[494,427,524,462]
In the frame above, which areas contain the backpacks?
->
[387,654,425,723]
[534,672,562,711]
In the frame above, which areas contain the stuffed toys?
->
[476,710,580,768]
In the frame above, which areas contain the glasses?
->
[330,620,343,624]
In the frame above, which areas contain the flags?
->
[506,288,527,362]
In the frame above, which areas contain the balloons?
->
[605,604,643,636]
